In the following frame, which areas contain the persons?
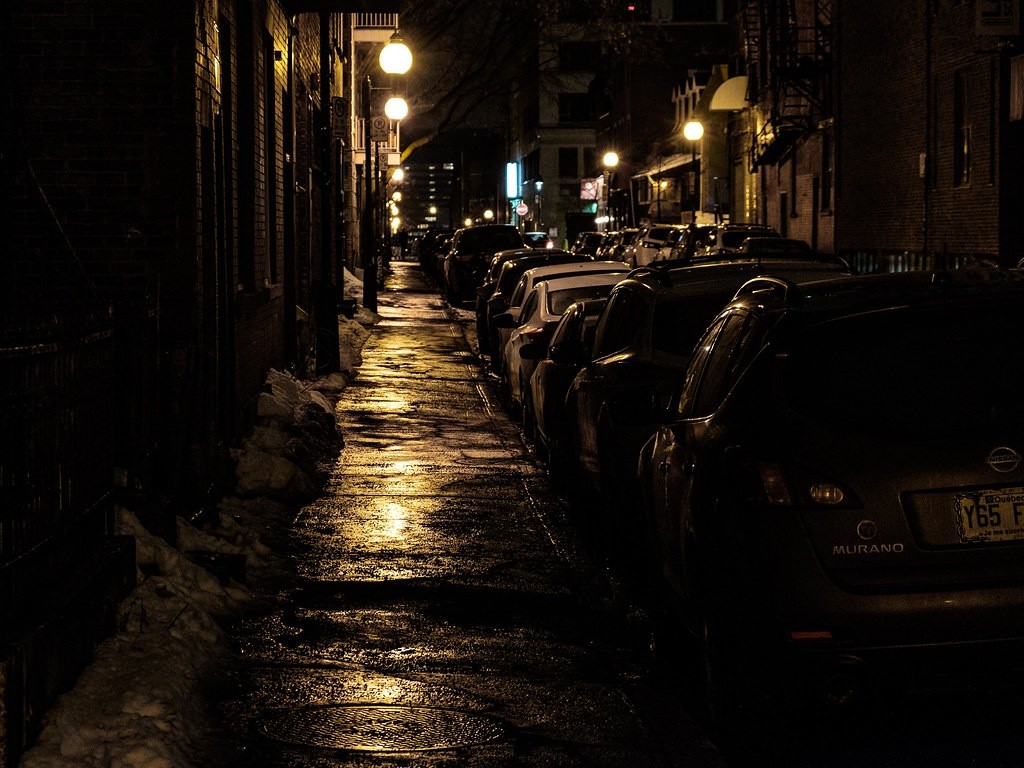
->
[391,226,411,260]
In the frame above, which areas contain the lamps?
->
[379,28,413,122]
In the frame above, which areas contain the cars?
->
[392,222,1024,744]
